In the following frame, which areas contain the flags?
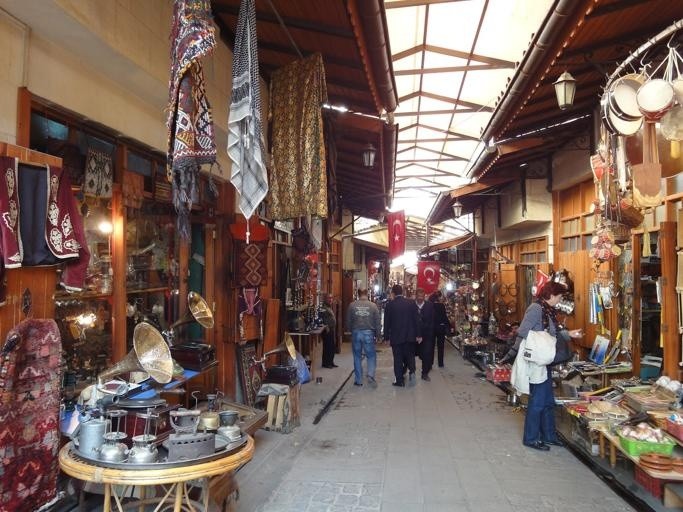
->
[417,261,441,295]
[386,209,405,259]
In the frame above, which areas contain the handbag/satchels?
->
[548,335,575,366]
[632,162,665,210]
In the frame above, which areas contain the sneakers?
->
[321,362,339,370]
[421,374,431,382]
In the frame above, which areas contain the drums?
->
[637,80,672,123]
[590,202,620,260]
[589,151,615,183]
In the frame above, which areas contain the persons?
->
[515,281,587,451]
[497,322,520,365]
[383,285,423,386]
[429,291,454,368]
[346,287,382,386]
[319,294,339,368]
[414,288,435,381]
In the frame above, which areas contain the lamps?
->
[361,132,376,165]
[452,198,462,218]
[552,58,578,109]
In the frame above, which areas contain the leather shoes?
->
[522,438,551,452]
[392,381,406,387]
[366,376,377,388]
[540,436,568,448]
[407,372,417,387]
[354,381,364,387]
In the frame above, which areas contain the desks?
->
[58,434,254,512]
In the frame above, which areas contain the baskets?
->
[607,204,646,229]
[607,220,633,245]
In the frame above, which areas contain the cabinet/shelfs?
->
[598,403,683,480]
